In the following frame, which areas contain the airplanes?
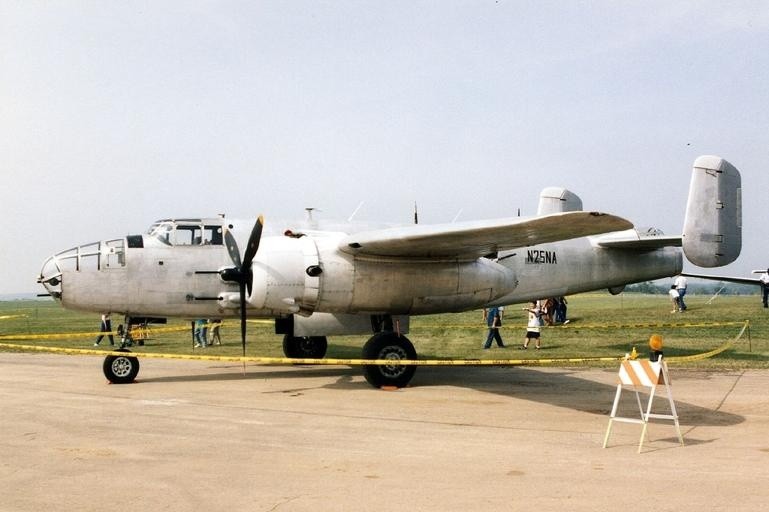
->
[34,154,742,389]
[679,269,768,308]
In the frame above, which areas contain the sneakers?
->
[671,306,687,313]
[543,320,570,326]
[518,345,540,350]
[194,343,221,348]
[94,342,99,346]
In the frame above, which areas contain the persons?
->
[759,268,769,307]
[674,275,689,310]
[207,319,224,345]
[94,314,118,347]
[518,301,541,351]
[540,296,571,327]
[194,320,207,348]
[482,306,505,349]
[669,285,683,313]
